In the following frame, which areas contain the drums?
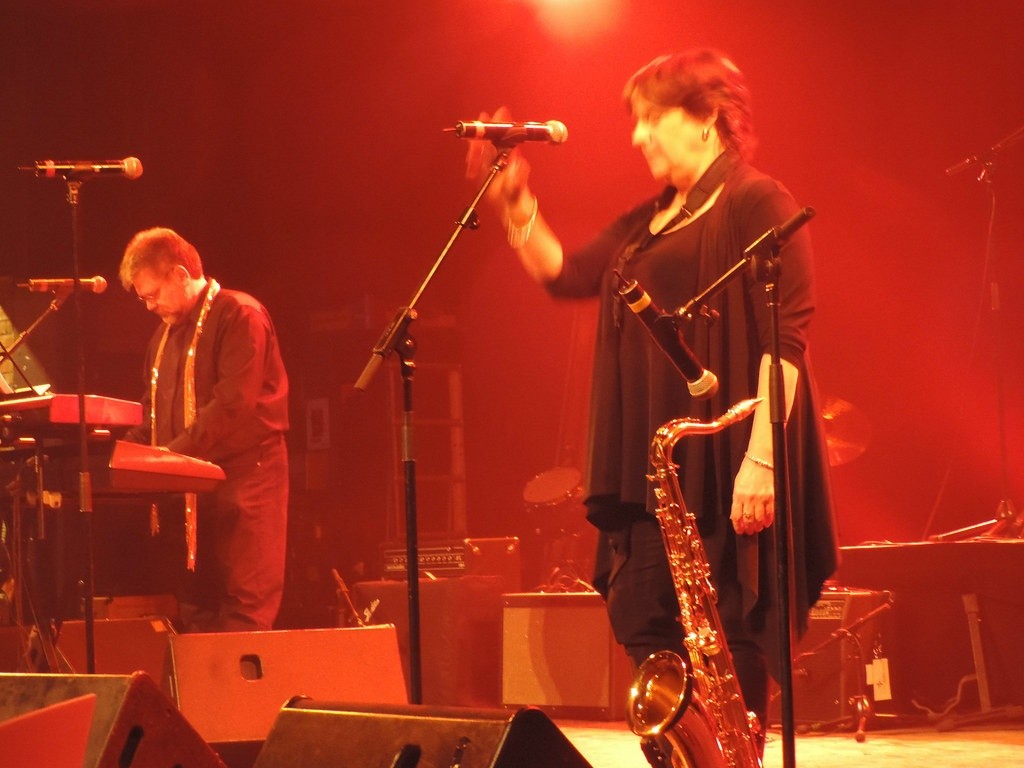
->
[522,465,587,537]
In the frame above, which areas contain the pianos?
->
[0,382,226,500]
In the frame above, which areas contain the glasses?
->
[136,267,173,305]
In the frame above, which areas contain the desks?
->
[502,591,639,722]
[836,539,1024,733]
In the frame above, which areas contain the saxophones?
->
[624,395,770,767]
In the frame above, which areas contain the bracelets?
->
[742,453,775,471]
[503,195,538,244]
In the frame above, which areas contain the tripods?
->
[792,592,943,742]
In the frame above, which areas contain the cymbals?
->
[818,393,874,468]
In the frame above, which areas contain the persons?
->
[120,227,291,633]
[467,49,835,757]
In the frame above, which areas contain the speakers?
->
[0,537,883,768]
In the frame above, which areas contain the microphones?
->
[612,268,719,401]
[18,157,144,179]
[792,669,807,682]
[443,120,569,144]
[17,276,108,295]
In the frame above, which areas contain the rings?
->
[743,511,755,519]
